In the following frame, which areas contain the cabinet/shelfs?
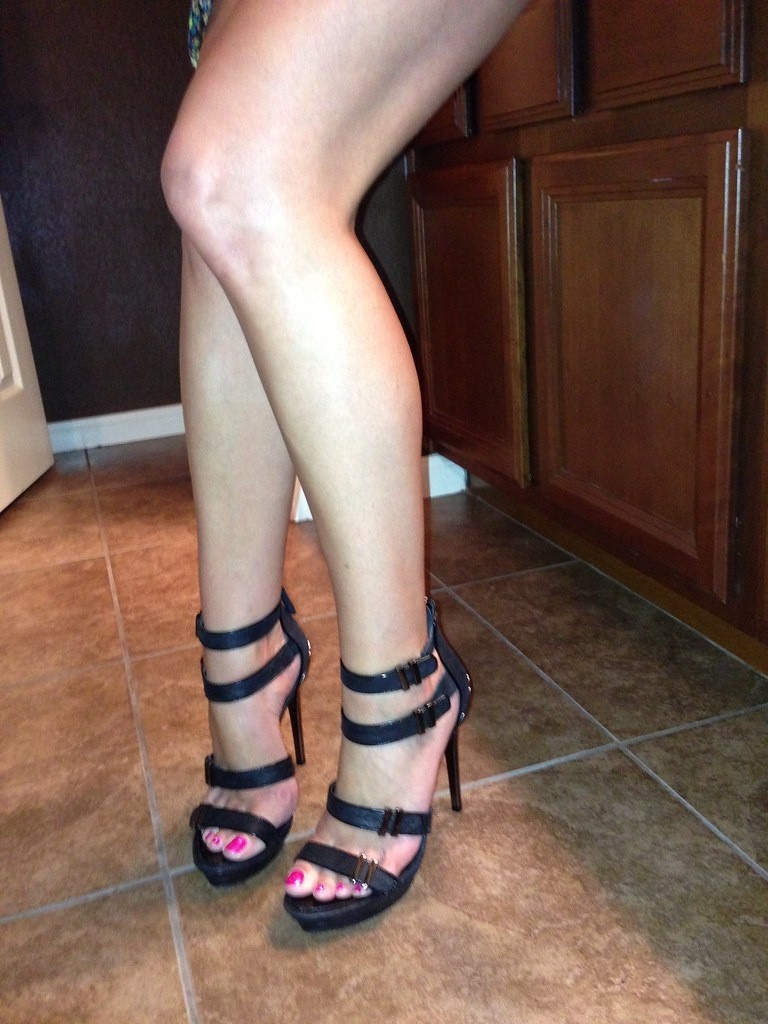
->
[404,0,768,683]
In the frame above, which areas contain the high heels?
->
[284,598,473,932]
[189,586,310,887]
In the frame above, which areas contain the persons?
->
[161,0,528,933]
